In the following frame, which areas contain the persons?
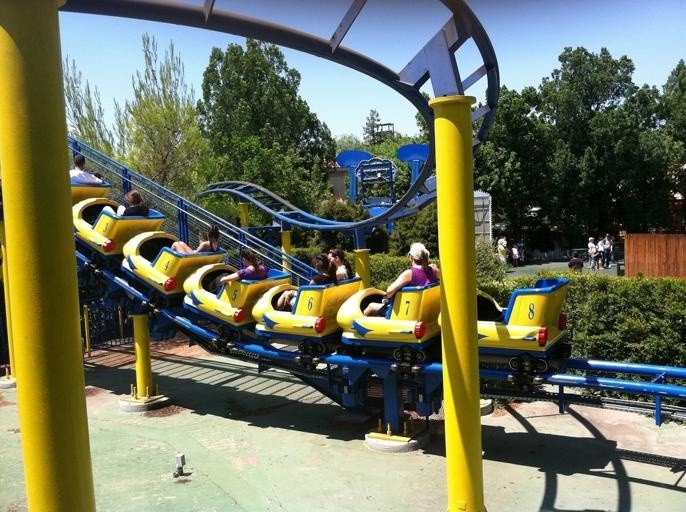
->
[601,232,611,269]
[171,224,223,254]
[275,254,337,311]
[102,190,150,216]
[208,250,268,294]
[588,237,596,270]
[597,238,605,270]
[68,153,105,183]
[327,248,357,281]
[497,239,509,267]
[511,244,520,267]
[591,248,599,268]
[363,242,439,316]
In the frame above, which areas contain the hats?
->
[408,243,429,259]
[589,237,595,241]
